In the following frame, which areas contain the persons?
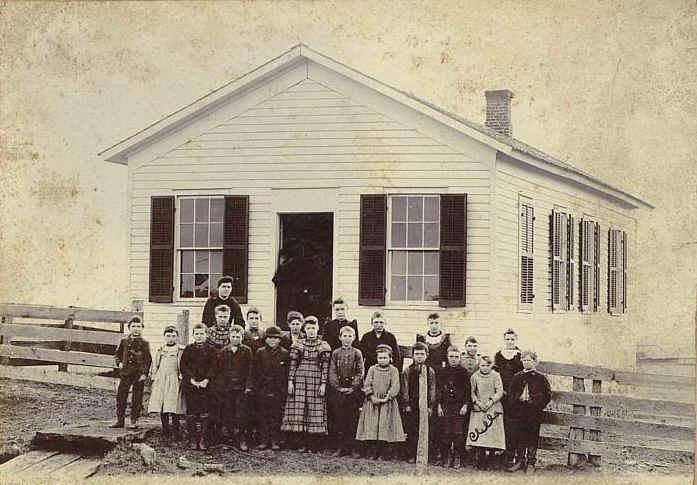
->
[201,275,245,331]
[110,316,152,428]
[272,238,302,331]
[148,297,552,476]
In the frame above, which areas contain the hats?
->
[266,311,318,339]
[377,345,392,351]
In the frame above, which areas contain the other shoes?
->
[162,436,328,457]
[331,439,536,476]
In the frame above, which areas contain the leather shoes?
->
[109,421,138,429]
